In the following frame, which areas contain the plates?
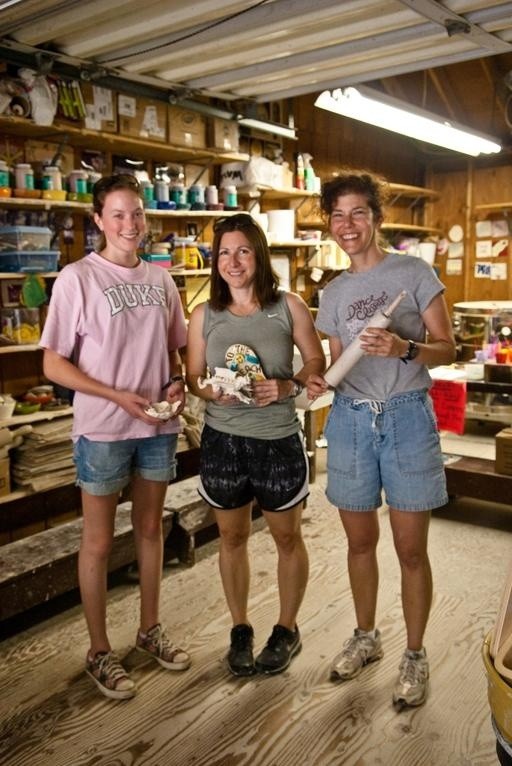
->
[253,208,295,240]
[144,402,183,419]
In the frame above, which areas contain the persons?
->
[313,173,457,711]
[39,173,190,702]
[185,213,327,677]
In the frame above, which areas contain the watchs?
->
[398,339,419,365]
[288,377,303,399]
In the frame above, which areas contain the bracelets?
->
[162,375,185,390]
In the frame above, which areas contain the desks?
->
[430,361,512,423]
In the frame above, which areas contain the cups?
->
[174,242,203,270]
[494,353,506,363]
[420,242,436,265]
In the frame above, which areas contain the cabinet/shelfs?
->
[298,180,443,236]
[251,186,331,248]
[0,116,251,504]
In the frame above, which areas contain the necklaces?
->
[236,304,257,319]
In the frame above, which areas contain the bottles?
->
[1,161,238,212]
[474,343,498,360]
[296,153,304,189]
[287,104,294,129]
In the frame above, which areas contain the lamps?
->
[312,69,504,158]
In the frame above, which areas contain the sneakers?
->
[332,629,381,680]
[86,647,138,700]
[226,624,253,676]
[393,648,428,705]
[255,623,302,675]
[138,624,192,670]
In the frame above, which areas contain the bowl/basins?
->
[1,396,16,420]
[23,393,56,404]
[16,400,41,414]
[44,399,71,410]
[299,230,322,240]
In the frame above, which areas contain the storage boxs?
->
[80,82,239,152]
